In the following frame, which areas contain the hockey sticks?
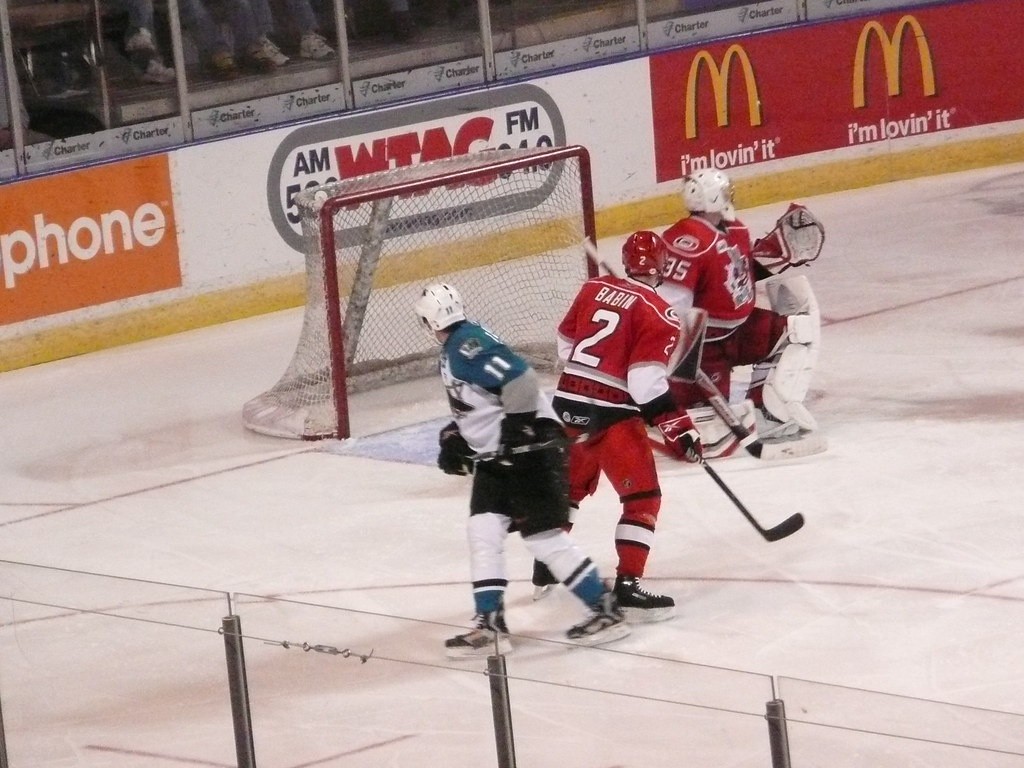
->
[581,237,829,461]
[692,448,803,544]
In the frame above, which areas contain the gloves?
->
[436,423,476,476]
[493,418,534,475]
[654,408,702,463]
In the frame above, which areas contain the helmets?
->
[414,282,466,346]
[622,230,667,285]
[683,167,736,223]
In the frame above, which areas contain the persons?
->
[653,168,828,460]
[412,283,631,658]
[529,231,701,624]
[119,0,416,84]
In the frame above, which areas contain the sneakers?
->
[532,557,562,600]
[444,600,513,658]
[611,575,676,623]
[566,591,631,647]
